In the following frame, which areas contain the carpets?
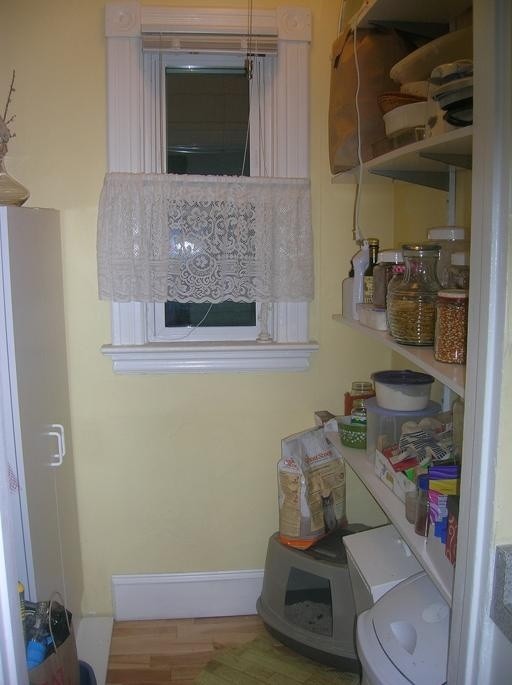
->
[189,628,359,685]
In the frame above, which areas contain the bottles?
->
[25,601,50,670]
[414,473,429,537]
[386,226,470,366]
[351,380,376,426]
[362,238,403,308]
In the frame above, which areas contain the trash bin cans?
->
[356,569,451,685]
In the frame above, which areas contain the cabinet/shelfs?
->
[322,3,471,612]
[1,205,85,637]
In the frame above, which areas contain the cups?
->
[406,491,419,524]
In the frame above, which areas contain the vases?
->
[0,142,31,203]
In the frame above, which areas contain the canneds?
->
[351,399,369,426]
[433,288,468,364]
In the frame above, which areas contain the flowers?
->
[0,66,18,157]
